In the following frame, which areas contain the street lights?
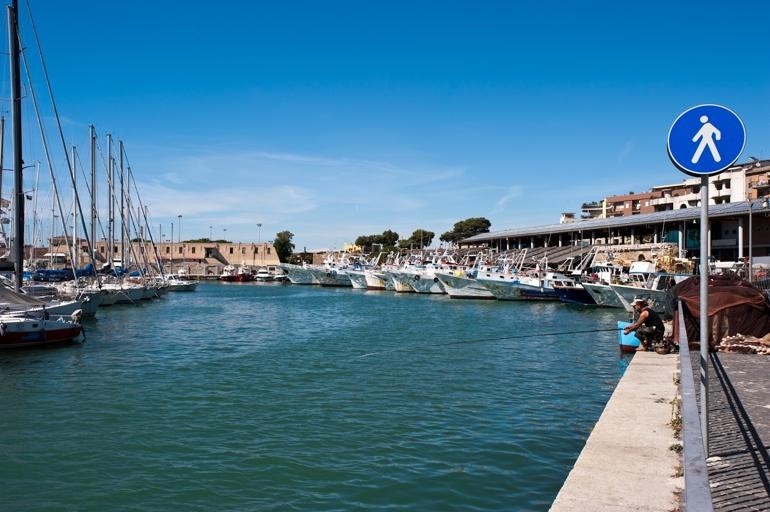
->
[208,225,213,240]
[223,227,227,241]
[257,223,263,241]
[745,196,754,285]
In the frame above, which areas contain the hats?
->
[630,298,644,306]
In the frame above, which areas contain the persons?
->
[624,299,665,351]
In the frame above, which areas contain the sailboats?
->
[0,1,200,348]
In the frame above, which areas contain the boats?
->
[617,293,660,353]
[219,241,692,311]
